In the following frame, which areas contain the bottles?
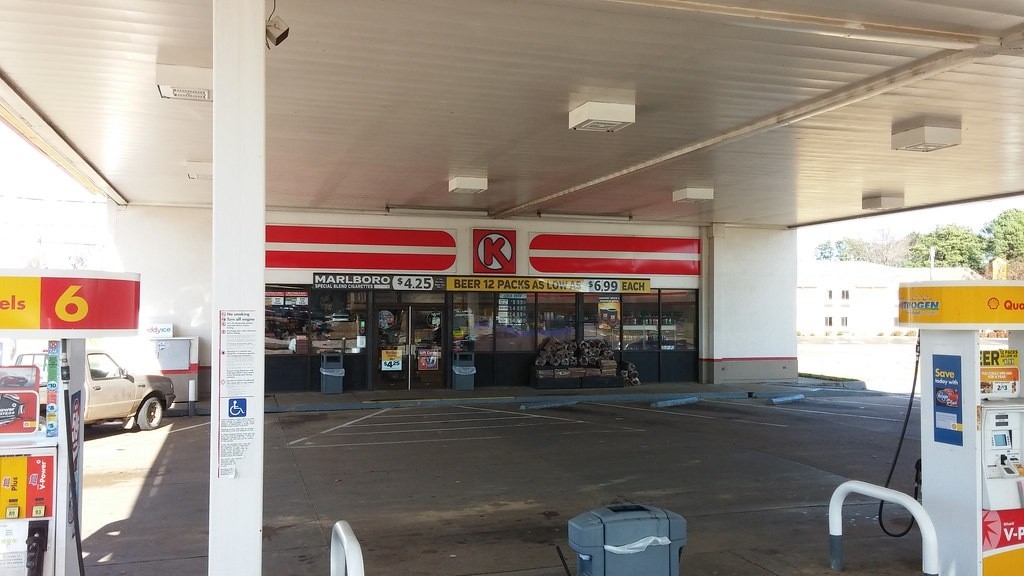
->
[622,312,684,325]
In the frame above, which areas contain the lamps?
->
[569,101,636,131]
[889,126,962,152]
[449,177,489,195]
[672,188,714,203]
[862,195,905,210]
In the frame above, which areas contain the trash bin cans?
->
[320,352,345,394]
[568,503,686,576]
[452,352,476,391]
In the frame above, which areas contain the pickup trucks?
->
[13,351,176,433]
